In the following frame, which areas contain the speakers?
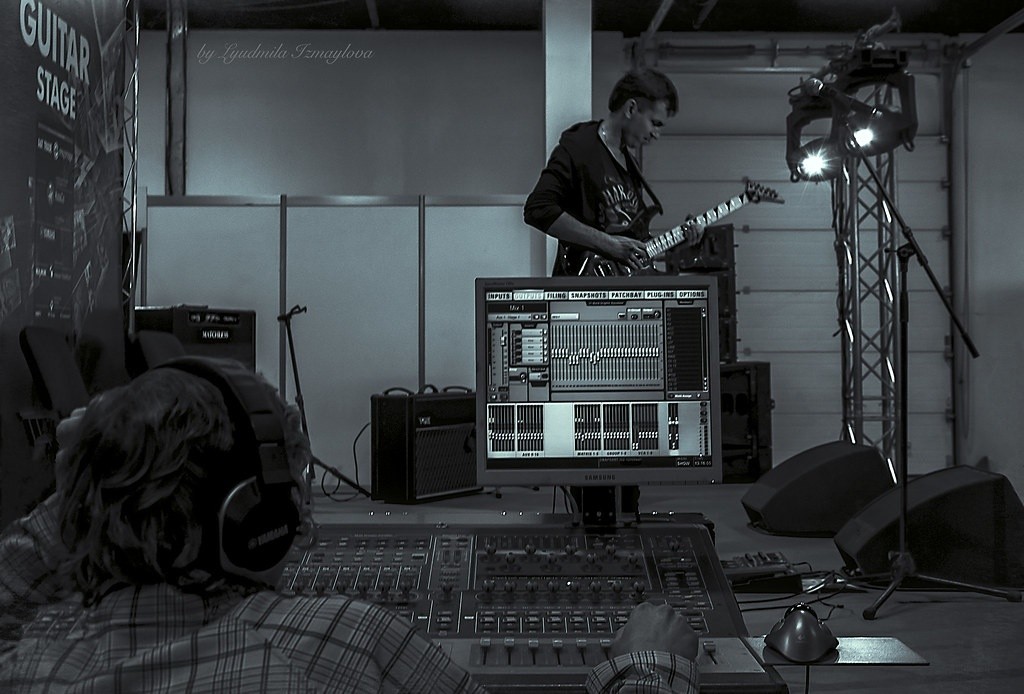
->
[721,359,772,484]
[370,384,484,506]
[832,464,1024,586]
[742,439,892,534]
[135,304,257,372]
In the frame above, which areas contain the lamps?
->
[786,69,921,182]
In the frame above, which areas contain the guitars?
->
[559,178,786,306]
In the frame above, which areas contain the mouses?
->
[763,602,839,662]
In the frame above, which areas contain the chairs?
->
[20,326,188,447]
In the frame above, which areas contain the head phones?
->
[149,355,301,581]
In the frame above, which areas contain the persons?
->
[0,357,700,693]
[524,67,703,514]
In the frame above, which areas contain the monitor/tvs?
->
[473,278,725,486]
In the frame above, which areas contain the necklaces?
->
[600,125,613,153]
